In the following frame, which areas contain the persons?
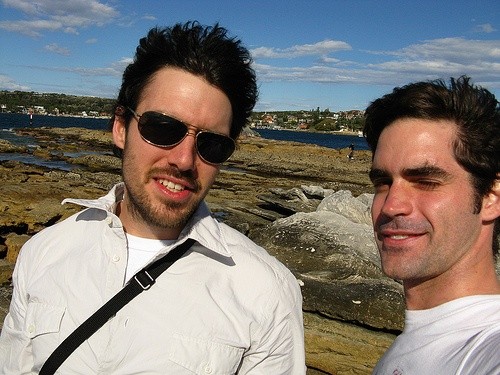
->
[361,73,499,375]
[0,21,307,375]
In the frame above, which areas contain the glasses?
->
[124,104,240,166]
